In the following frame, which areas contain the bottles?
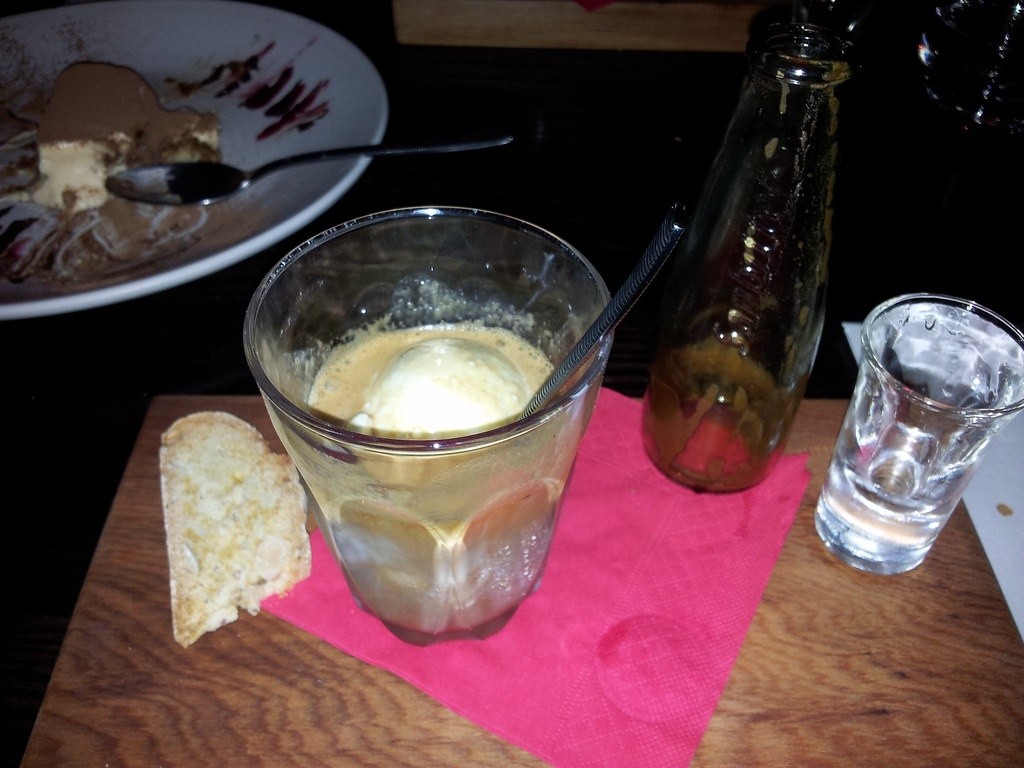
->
[641,7,865,495]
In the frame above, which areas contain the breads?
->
[159,410,312,647]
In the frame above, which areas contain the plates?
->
[0,0,388,319]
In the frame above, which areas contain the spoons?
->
[104,132,514,207]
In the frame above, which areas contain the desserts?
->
[32,62,218,212]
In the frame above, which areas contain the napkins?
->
[260,389,816,768]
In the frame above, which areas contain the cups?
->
[242,207,617,648]
[814,291,1024,576]
